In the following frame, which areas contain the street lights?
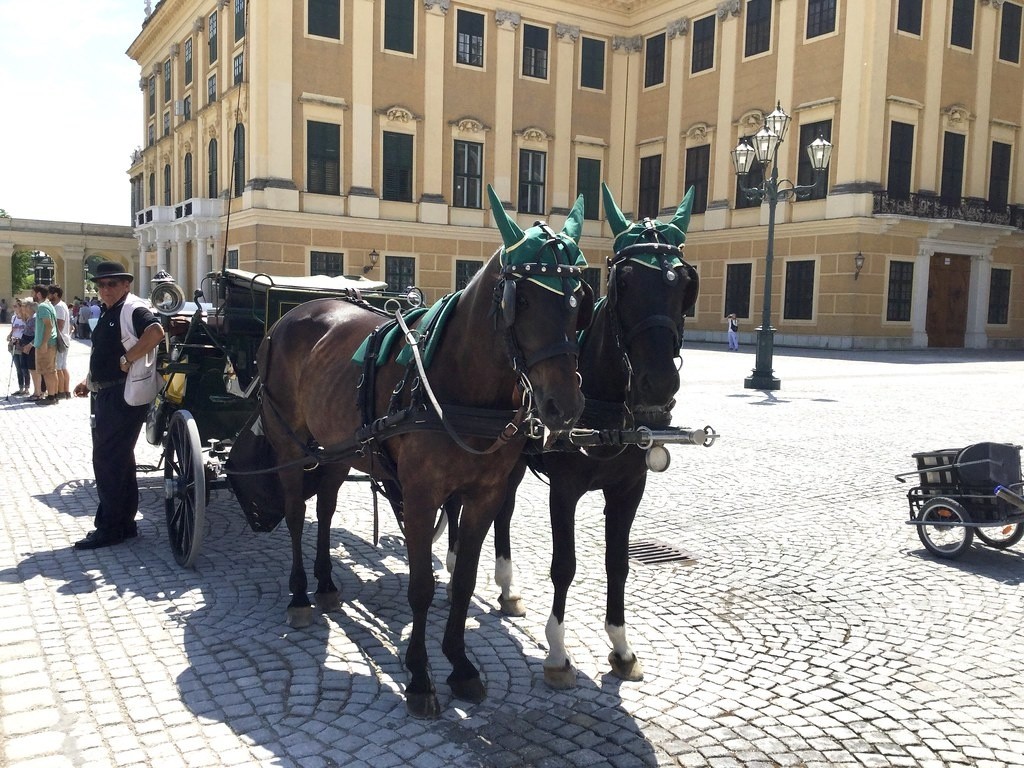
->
[730,99,834,390]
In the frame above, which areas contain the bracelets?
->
[29,342,34,346]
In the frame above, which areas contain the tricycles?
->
[905,442,1024,559]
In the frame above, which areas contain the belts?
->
[97,378,125,389]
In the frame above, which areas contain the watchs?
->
[121,355,132,366]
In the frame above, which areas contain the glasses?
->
[96,281,121,287]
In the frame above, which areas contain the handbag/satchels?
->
[8,337,22,354]
[56,329,68,352]
[731,324,738,332]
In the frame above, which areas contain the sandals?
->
[23,394,43,402]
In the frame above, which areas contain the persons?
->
[727,314,739,352]
[73,263,164,549]
[0,284,107,405]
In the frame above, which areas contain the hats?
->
[90,261,134,283]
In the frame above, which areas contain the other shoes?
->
[18,390,30,396]
[35,393,71,406]
[11,390,22,396]
[728,348,738,352]
[88,521,138,538]
[75,527,124,549]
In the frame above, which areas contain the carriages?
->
[147,182,721,720]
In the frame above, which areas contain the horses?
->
[257,179,700,718]
